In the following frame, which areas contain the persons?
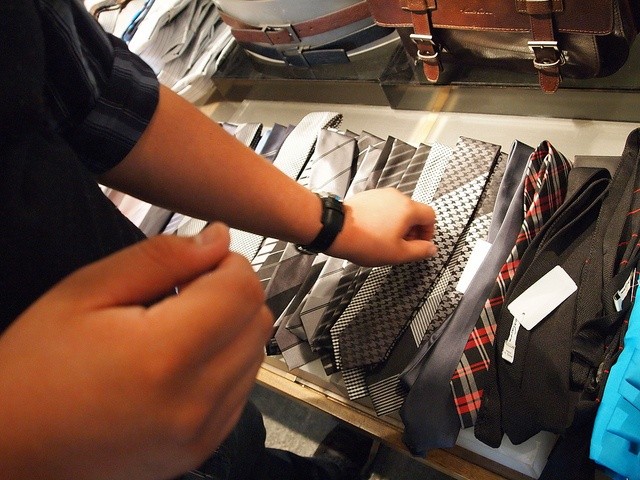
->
[0,0,439,480]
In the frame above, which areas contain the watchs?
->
[295,191,345,256]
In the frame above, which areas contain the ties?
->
[98,185,209,239]
[212,111,639,458]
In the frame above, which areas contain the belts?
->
[217,1,372,46]
[240,23,395,69]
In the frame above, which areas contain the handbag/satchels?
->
[366,1,638,94]
